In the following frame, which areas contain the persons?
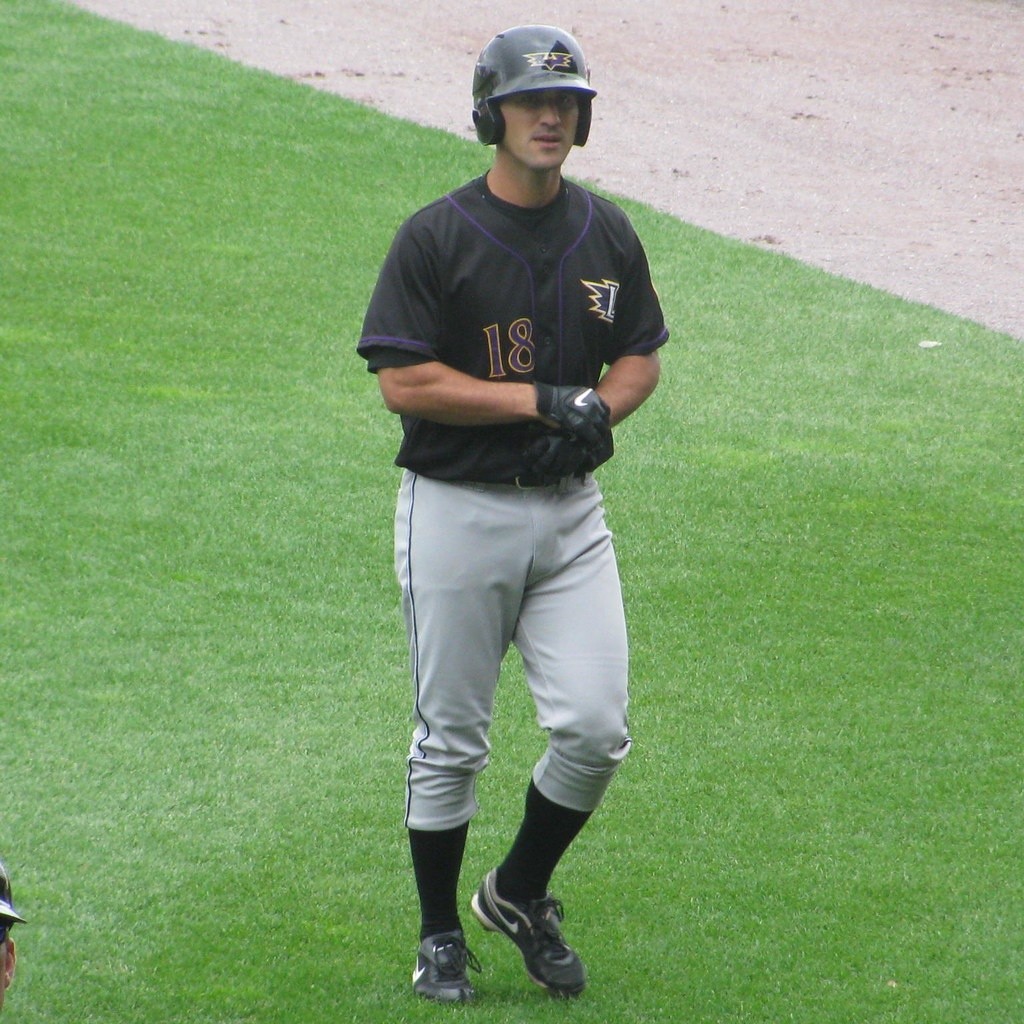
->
[354,22,669,1005]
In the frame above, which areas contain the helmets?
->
[472,24,597,146]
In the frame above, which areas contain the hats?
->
[0,864,27,923]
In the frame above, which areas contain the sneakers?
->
[470,869,588,997]
[413,926,484,1001]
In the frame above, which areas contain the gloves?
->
[534,384,612,452]
[513,434,589,494]
